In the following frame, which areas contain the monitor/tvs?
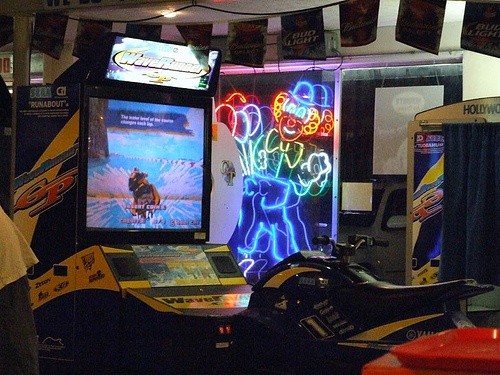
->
[340,182,372,211]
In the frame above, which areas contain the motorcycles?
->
[229,235,495,375]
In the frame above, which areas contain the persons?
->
[0,207,39,374]
